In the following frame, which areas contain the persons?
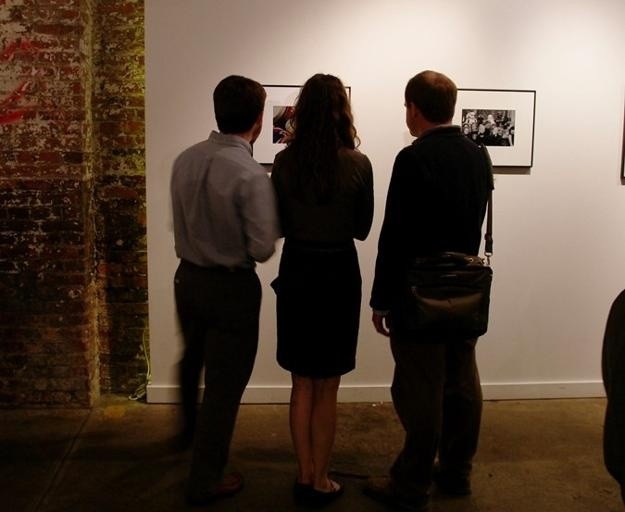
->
[463,109,514,146]
[269,71,377,505]
[356,67,493,510]
[167,74,279,506]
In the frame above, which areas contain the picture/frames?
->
[252,84,351,165]
[450,88,536,168]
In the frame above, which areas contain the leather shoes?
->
[294,479,345,501]
[365,474,429,504]
[431,458,473,496]
[210,471,244,497]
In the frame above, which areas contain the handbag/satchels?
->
[408,251,493,340]
